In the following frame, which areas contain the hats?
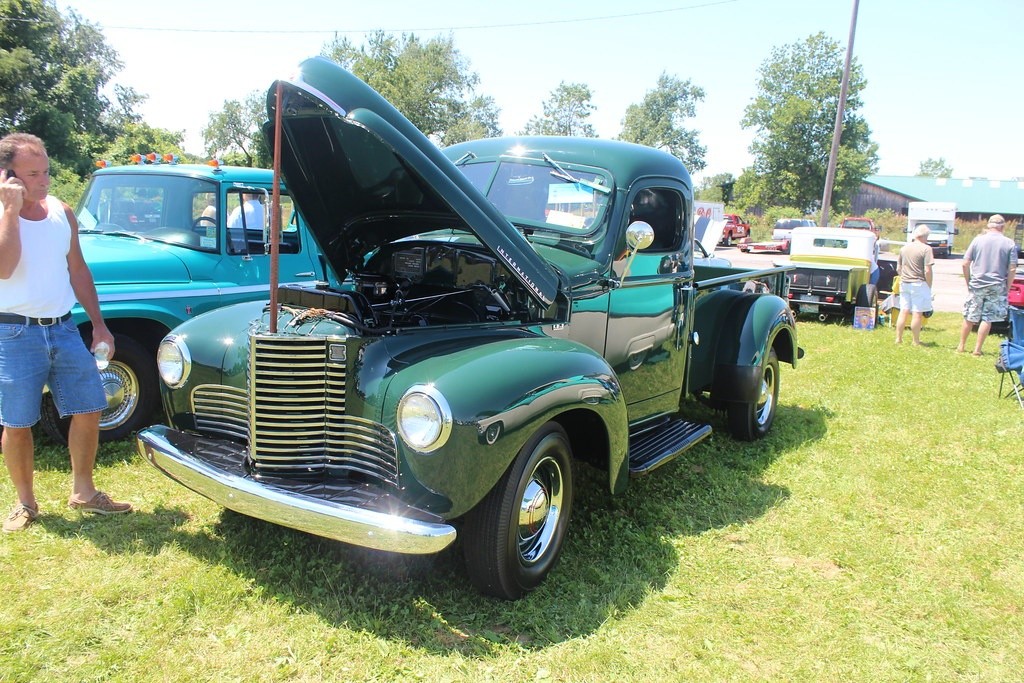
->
[988,214,1005,224]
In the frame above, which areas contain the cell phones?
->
[6,170,16,179]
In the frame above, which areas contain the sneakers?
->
[2,502,40,534]
[68,491,133,516]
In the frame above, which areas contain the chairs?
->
[994,304,1024,409]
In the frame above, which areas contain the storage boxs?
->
[853,307,877,330]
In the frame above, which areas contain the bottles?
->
[94,341,110,369]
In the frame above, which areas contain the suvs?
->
[772,219,818,244]
[722,214,750,247]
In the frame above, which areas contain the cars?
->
[1007,258,1024,307]
[34,152,340,447]
[841,218,879,242]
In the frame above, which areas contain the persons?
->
[201,193,228,227]
[957,214,1017,355]
[227,193,264,230]
[0,133,131,533]
[895,224,935,345]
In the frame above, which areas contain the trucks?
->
[904,200,958,259]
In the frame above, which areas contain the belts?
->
[0,311,71,327]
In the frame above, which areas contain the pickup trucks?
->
[137,52,804,601]
[773,225,906,324]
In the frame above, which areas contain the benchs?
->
[230,239,290,254]
